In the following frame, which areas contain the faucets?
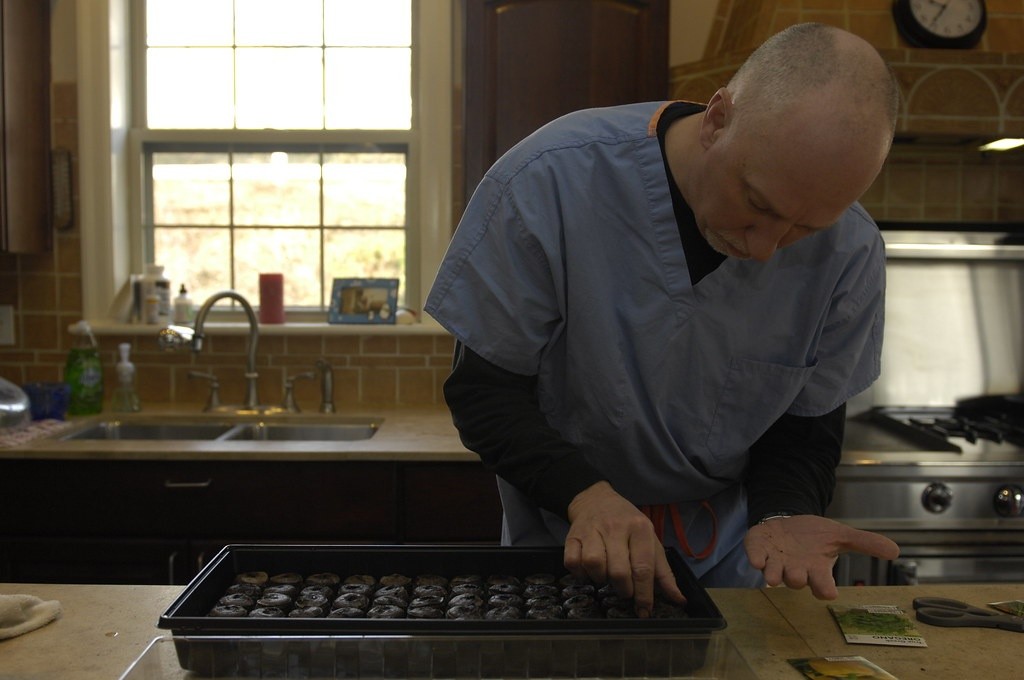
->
[190,290,259,412]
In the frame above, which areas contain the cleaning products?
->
[57,321,106,419]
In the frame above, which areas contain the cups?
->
[259,273,285,325]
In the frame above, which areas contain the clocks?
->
[892,0,988,50]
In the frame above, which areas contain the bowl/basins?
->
[24,382,73,422]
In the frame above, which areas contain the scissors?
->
[913,595,1024,633]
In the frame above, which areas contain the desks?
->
[0,583,1024,679]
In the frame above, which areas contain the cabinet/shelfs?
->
[462,0,671,217]
[0,0,52,256]
[0,458,503,586]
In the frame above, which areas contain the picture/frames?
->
[329,278,400,323]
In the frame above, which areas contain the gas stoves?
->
[821,392,1024,530]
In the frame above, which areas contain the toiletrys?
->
[101,340,147,416]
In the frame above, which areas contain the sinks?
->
[56,412,236,445]
[220,413,386,442]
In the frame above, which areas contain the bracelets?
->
[765,512,792,523]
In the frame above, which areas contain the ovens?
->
[833,531,1024,586]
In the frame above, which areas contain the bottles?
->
[129,265,193,324]
[113,343,137,413]
[64,319,104,416]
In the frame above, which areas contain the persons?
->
[424,23,900,612]
